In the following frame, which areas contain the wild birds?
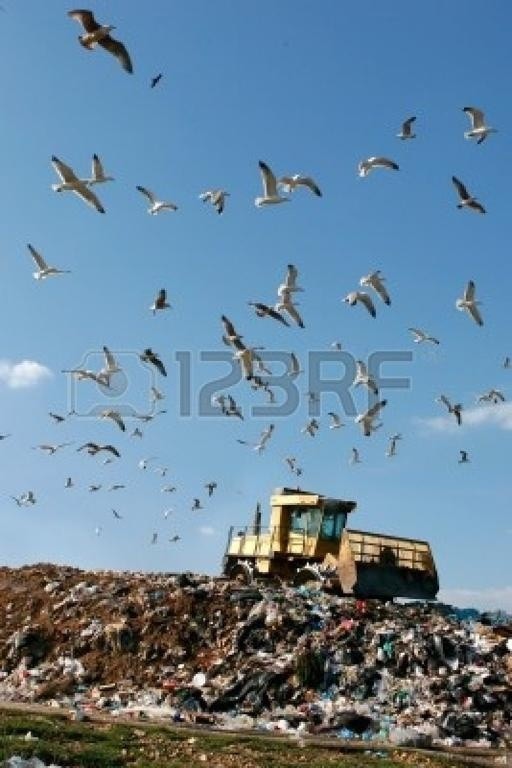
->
[0,107,512,544]
[67,9,134,75]
[150,73,164,89]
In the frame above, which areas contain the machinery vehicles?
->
[223,485,442,602]
